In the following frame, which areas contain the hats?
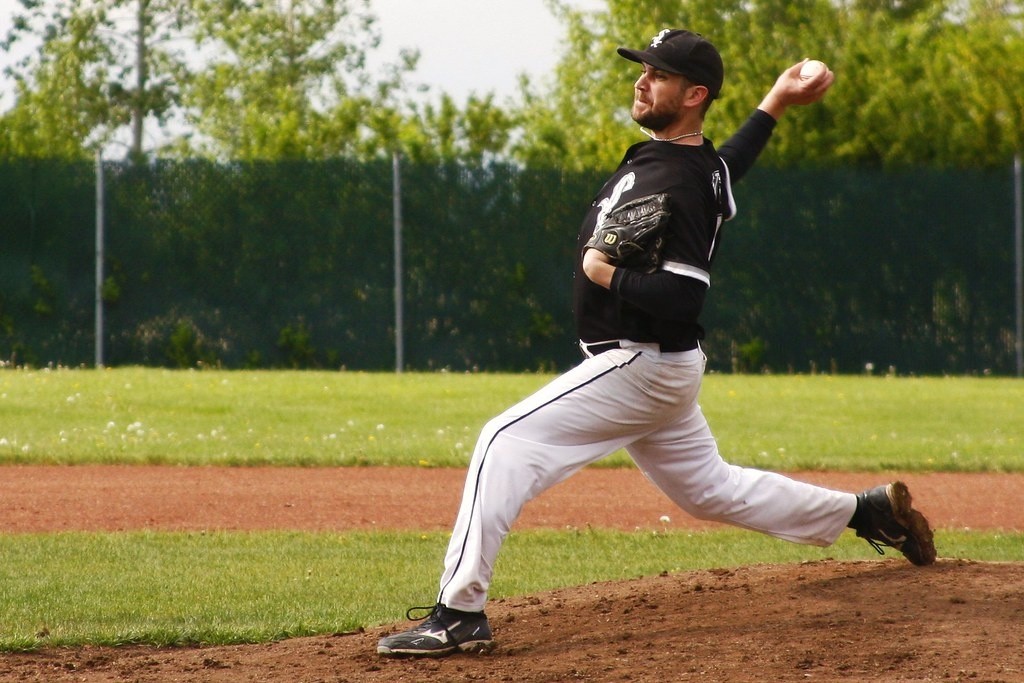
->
[617,29,723,91]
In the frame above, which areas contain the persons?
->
[377,28,937,654]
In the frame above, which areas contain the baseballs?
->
[800,59,828,84]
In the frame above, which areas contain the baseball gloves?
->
[581,187,668,266]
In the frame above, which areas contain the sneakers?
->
[375,604,494,655]
[856,481,937,567]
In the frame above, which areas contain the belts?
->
[579,337,701,359]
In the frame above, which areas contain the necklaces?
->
[640,127,703,141]
